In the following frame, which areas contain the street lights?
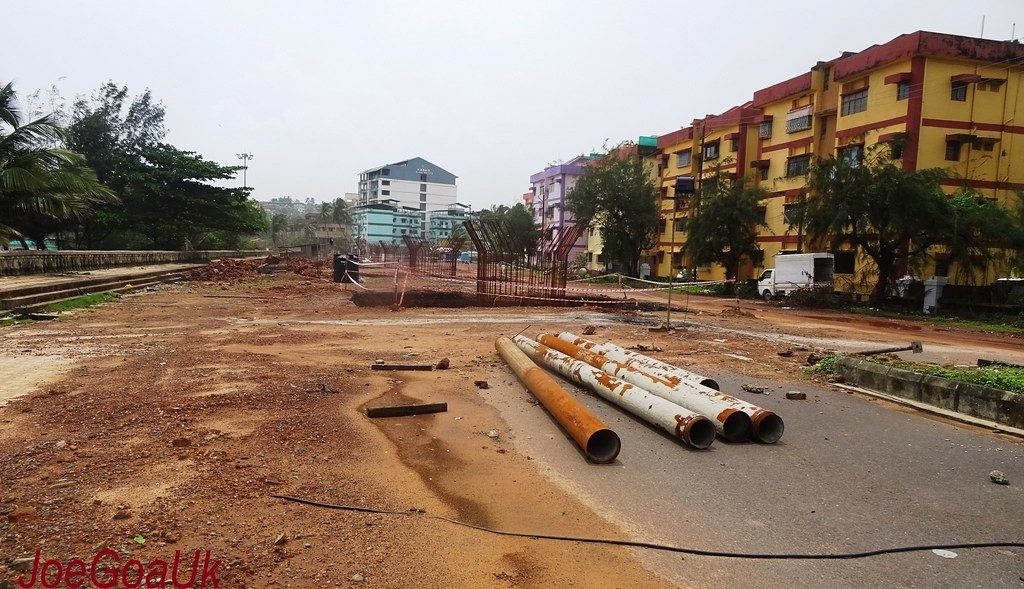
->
[238,153,254,188]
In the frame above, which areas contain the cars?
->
[677,270,699,279]
[442,248,461,259]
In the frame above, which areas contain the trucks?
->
[757,252,834,301]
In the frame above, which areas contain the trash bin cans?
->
[333,253,360,283]
